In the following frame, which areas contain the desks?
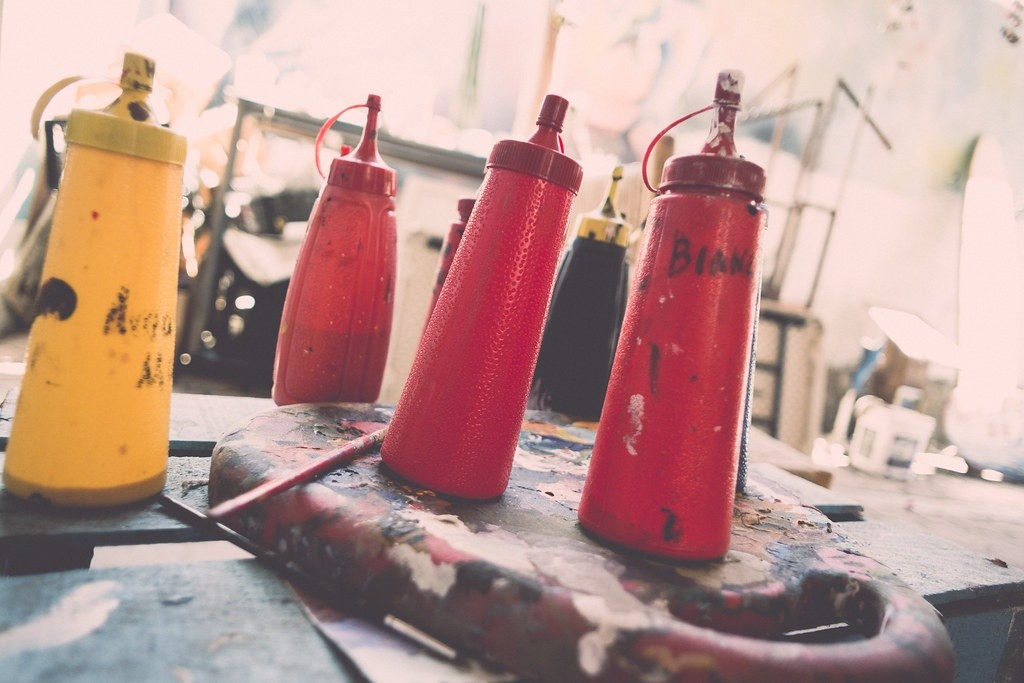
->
[1,371,1024,683]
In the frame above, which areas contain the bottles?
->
[380,94,585,500]
[524,166,632,422]
[578,68,767,563]
[6,53,185,506]
[271,94,398,407]
